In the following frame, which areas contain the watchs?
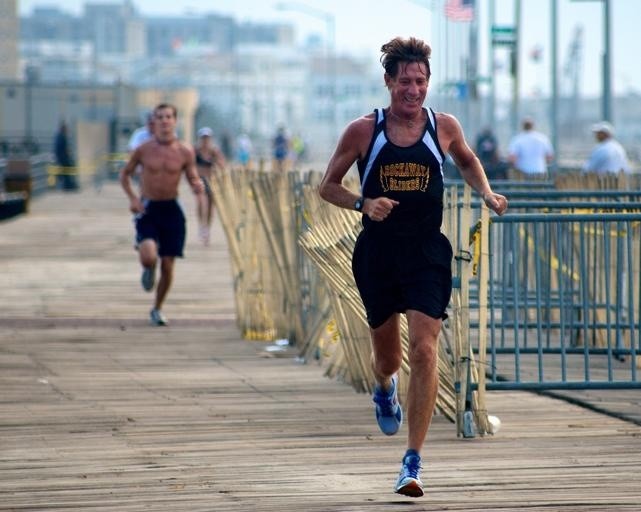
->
[353,195,364,212]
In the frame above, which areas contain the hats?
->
[197,127,213,138]
[591,121,614,137]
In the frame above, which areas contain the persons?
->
[126,110,180,185]
[235,132,253,170]
[194,126,227,244]
[272,126,288,173]
[54,121,81,191]
[119,103,205,327]
[473,124,499,159]
[582,119,632,173]
[502,116,557,176]
[318,33,507,501]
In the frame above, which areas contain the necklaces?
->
[389,110,421,129]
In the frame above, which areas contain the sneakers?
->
[149,308,167,327]
[197,227,213,247]
[373,374,403,436]
[141,258,158,292]
[392,458,425,497]
